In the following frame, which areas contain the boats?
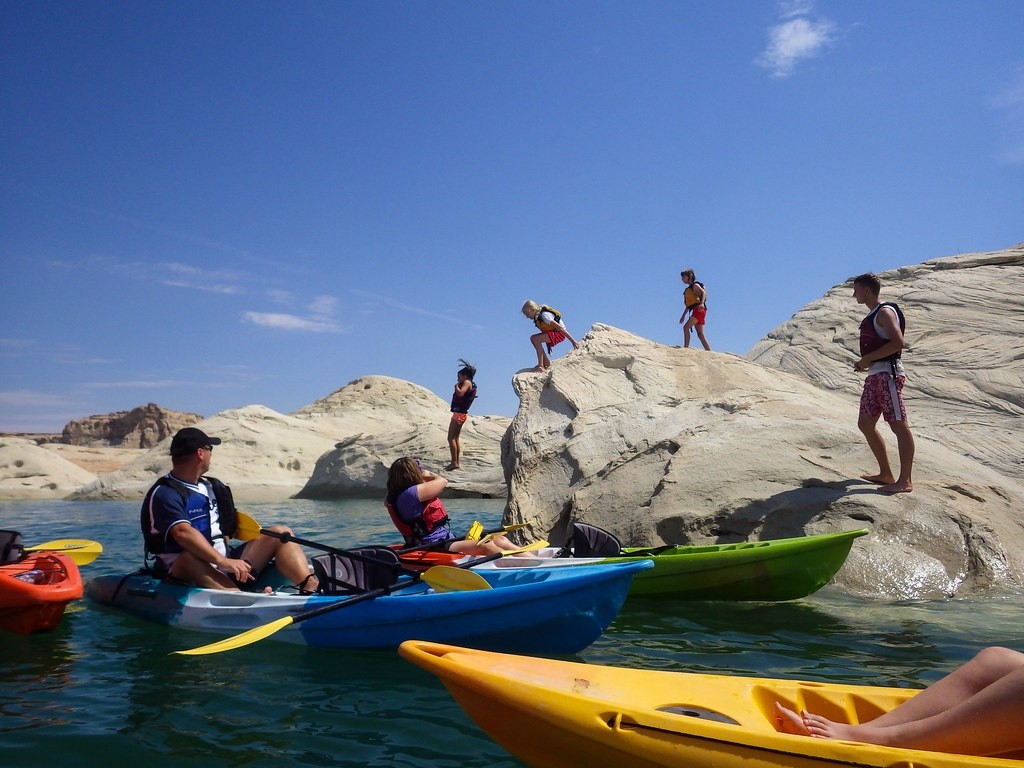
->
[384,527,870,600]
[0,548,83,617]
[396,638,1024,767]
[82,560,653,653]
[23,536,103,566]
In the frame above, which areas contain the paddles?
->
[395,521,533,556]
[237,512,493,594]
[169,539,549,657]
[24,539,103,566]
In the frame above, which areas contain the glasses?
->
[202,446,214,452]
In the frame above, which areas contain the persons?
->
[853,273,915,493]
[680,270,711,351]
[443,359,478,470]
[385,458,542,560]
[774,644,1024,755]
[521,300,579,371]
[141,428,319,596]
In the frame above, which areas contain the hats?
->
[169,427,221,462]
